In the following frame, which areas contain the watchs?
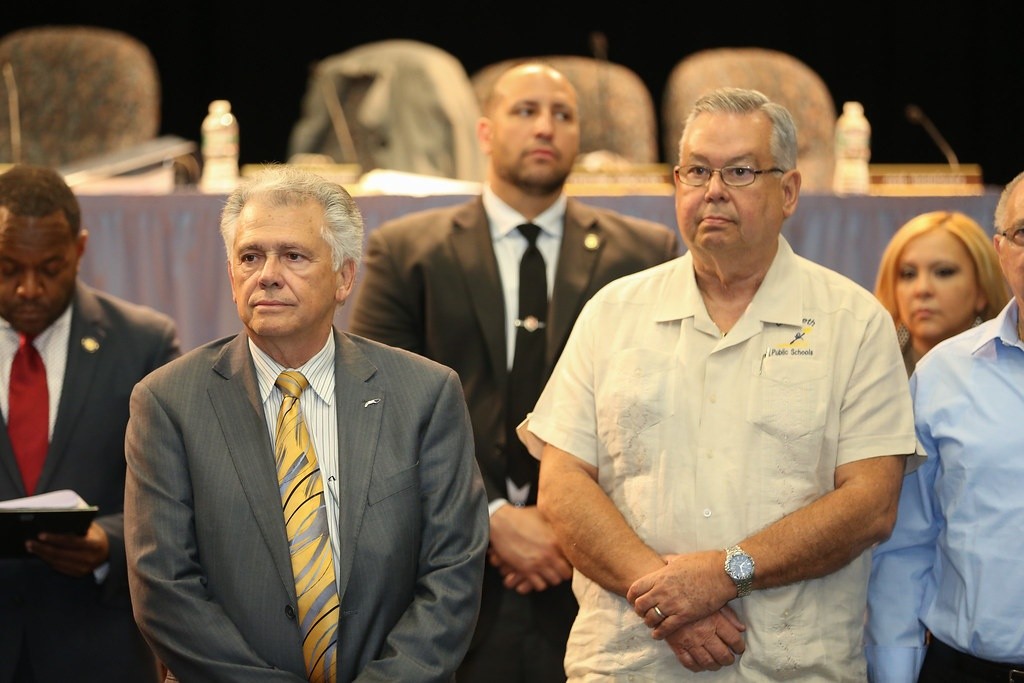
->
[724,545,755,600]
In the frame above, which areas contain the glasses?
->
[675,163,785,188]
[1002,228,1024,246]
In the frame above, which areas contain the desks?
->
[71,188,1006,355]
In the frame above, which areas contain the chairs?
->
[1,27,835,168]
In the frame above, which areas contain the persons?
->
[347,65,685,683]
[124,166,490,683]
[0,166,181,683]
[867,171,1024,683]
[514,88,929,683]
[873,210,1013,380]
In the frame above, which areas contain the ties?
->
[508,224,549,490]
[272,371,340,683]
[7,329,49,497]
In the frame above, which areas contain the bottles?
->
[834,102,871,195]
[200,101,240,193]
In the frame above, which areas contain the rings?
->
[654,605,665,620]
